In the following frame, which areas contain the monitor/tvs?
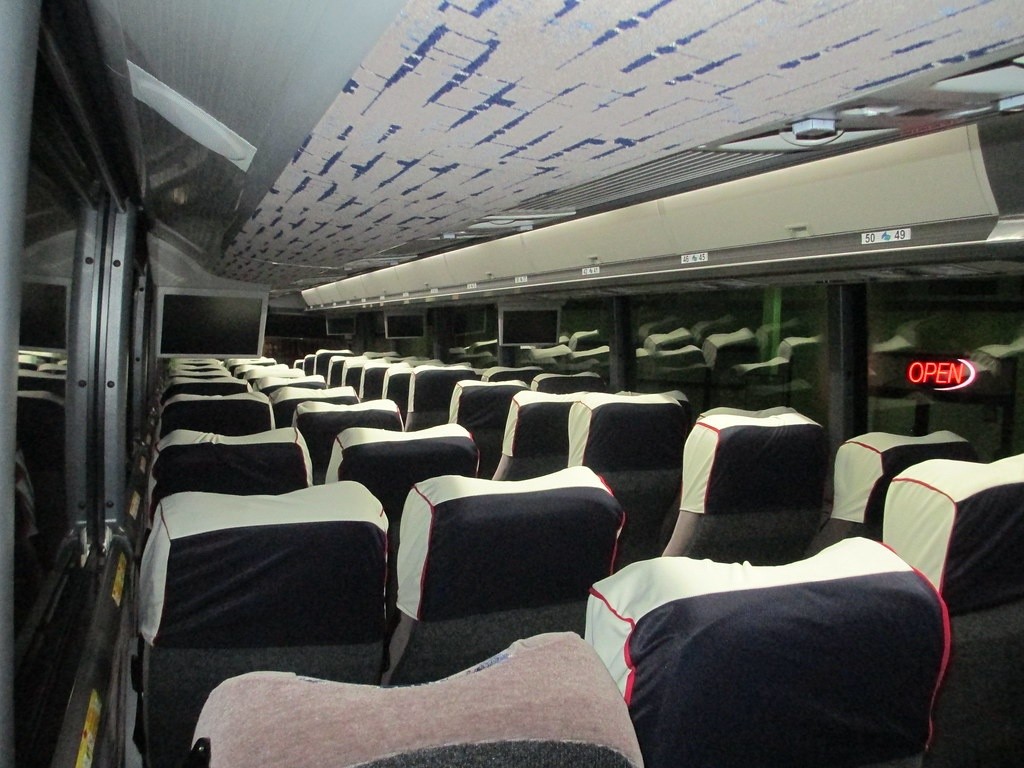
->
[450,305,487,338]
[497,304,561,346]
[326,314,356,336]
[18,273,72,353]
[385,310,428,340]
[155,286,270,360]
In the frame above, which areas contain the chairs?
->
[14,343,67,631]
[128,344,1023,768]
[448,313,1022,464]
